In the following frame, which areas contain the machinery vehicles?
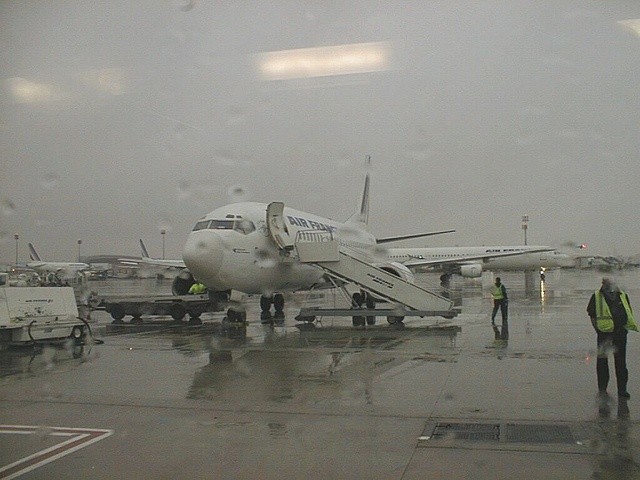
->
[86,267,227,320]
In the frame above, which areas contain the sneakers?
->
[618,392,630,397]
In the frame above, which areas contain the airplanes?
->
[182,152,569,326]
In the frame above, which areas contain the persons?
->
[490,277,508,325]
[587,275,640,398]
[187,281,207,294]
